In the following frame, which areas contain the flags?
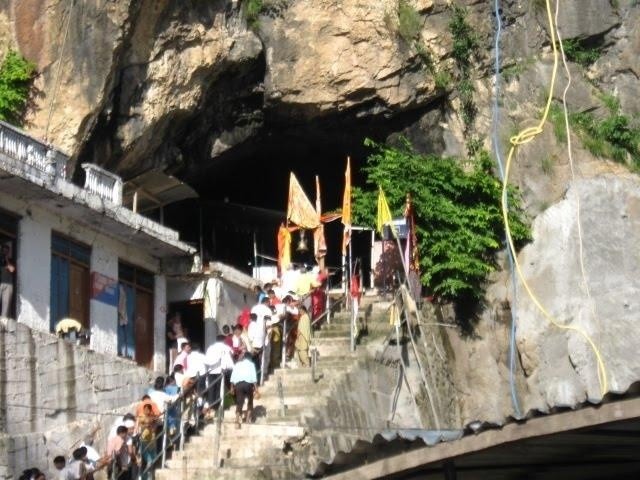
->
[277,156,420,296]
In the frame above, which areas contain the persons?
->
[206,264,318,429]
[0,245,15,317]
[103,314,221,479]
[19,442,111,479]
